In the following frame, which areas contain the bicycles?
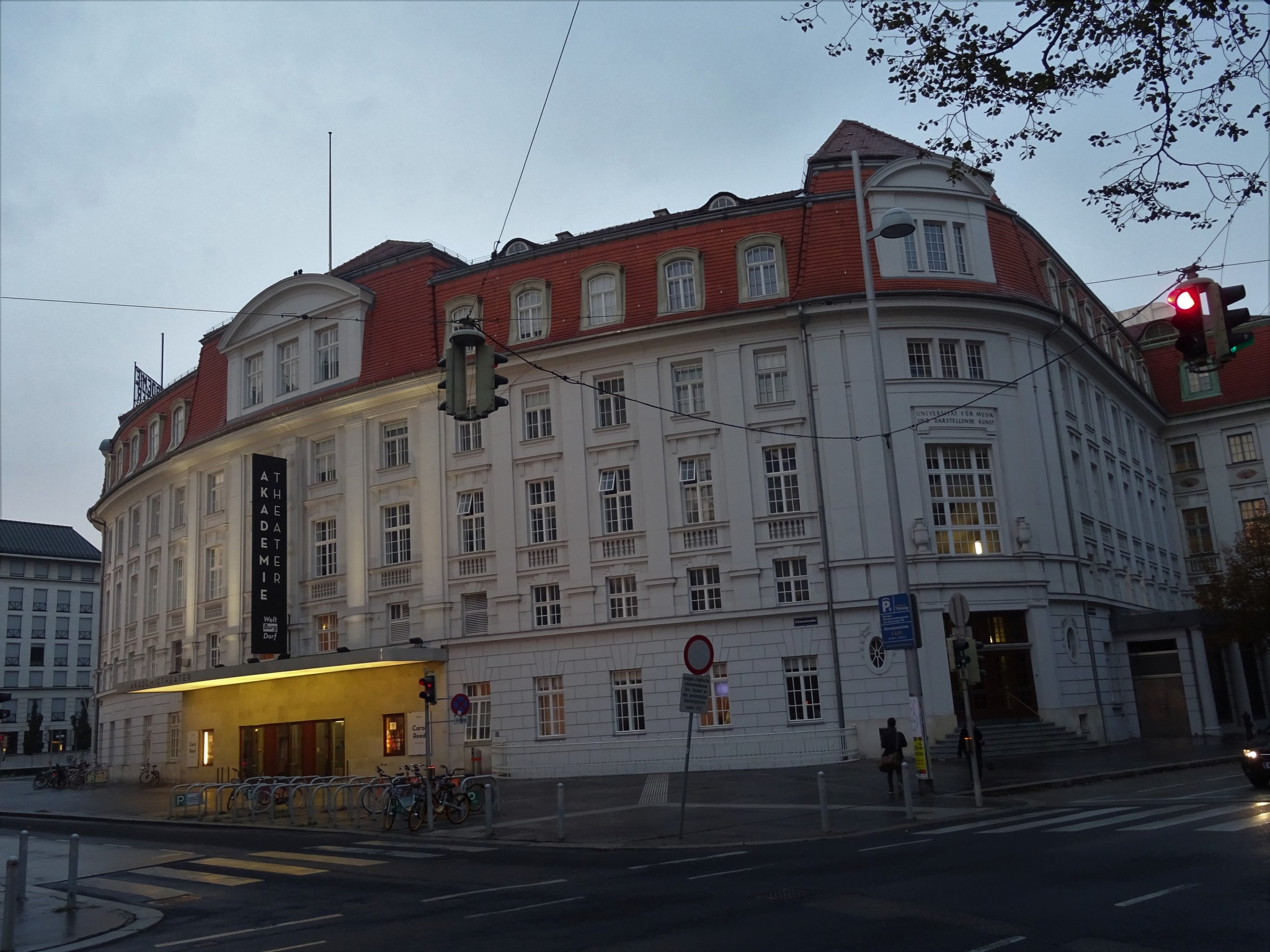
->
[32,759,110,790]
[139,758,160,785]
[362,763,486,832]
[226,767,304,813]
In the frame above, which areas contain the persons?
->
[1242,709,1253,741]
[958,719,986,789]
[881,717,907,801]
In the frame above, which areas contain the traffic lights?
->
[1205,282,1254,363]
[474,342,509,419]
[418,674,437,706]
[946,637,971,673]
[1166,283,1210,369]
[437,347,468,419]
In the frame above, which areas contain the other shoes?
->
[888,789,894,794]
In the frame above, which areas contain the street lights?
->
[849,148,937,794]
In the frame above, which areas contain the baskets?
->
[390,785,404,796]
[413,787,424,796]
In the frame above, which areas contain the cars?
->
[1240,720,1270,790]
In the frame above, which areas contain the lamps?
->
[247,658,260,663]
[337,647,350,653]
[214,664,225,667]
[278,654,290,660]
[409,637,423,648]
[169,671,178,674]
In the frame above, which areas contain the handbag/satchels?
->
[878,752,900,773]
